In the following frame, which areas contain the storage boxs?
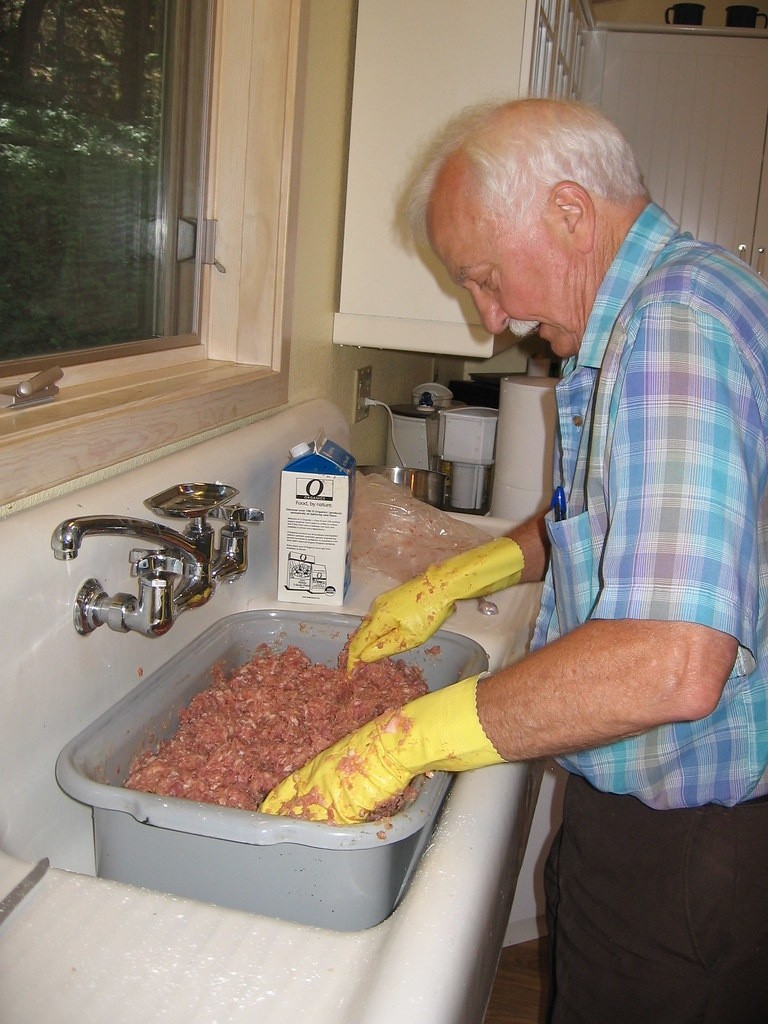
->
[55,611,489,925]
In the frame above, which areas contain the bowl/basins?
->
[353,465,449,511]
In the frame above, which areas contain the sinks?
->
[0,588,496,935]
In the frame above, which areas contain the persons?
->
[256,98,767,1023]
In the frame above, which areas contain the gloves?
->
[258,672,511,827]
[344,538,525,676]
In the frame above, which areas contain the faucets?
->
[51,503,265,638]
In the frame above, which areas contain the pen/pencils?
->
[548,485,567,524]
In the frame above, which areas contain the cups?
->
[666,3,705,24]
[724,4,767,27]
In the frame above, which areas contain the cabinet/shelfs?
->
[332,0,596,357]
[580,20,768,283]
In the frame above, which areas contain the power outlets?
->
[354,364,371,422]
